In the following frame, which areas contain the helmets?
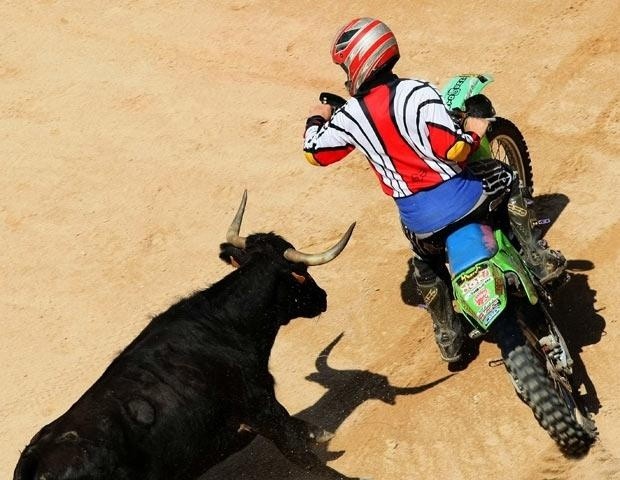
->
[330,16,402,97]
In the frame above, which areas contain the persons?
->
[302,17,569,363]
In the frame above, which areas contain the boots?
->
[507,191,568,285]
[410,269,470,361]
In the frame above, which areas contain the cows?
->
[12,189,357,480]
